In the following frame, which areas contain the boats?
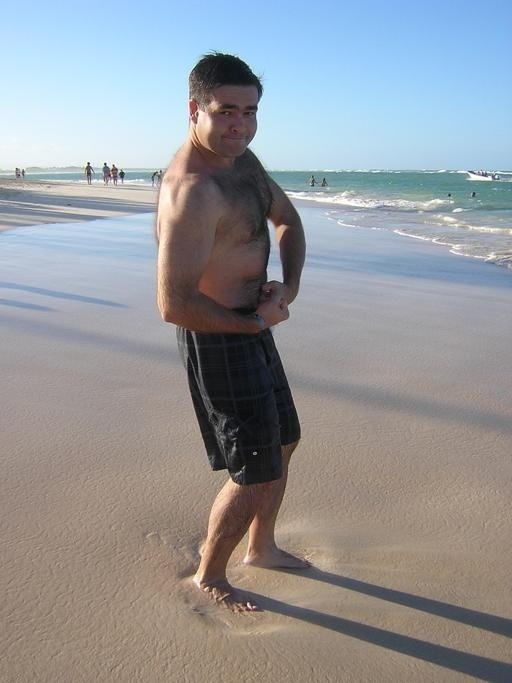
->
[467,170,497,181]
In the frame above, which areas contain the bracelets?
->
[247,312,265,333]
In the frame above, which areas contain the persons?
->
[156,168,163,188]
[319,177,328,187]
[470,191,476,197]
[154,49,309,615]
[110,163,119,186]
[102,161,110,186]
[21,168,26,179]
[151,170,158,188]
[308,175,317,186]
[119,168,126,184]
[84,161,95,185]
[15,167,20,179]
